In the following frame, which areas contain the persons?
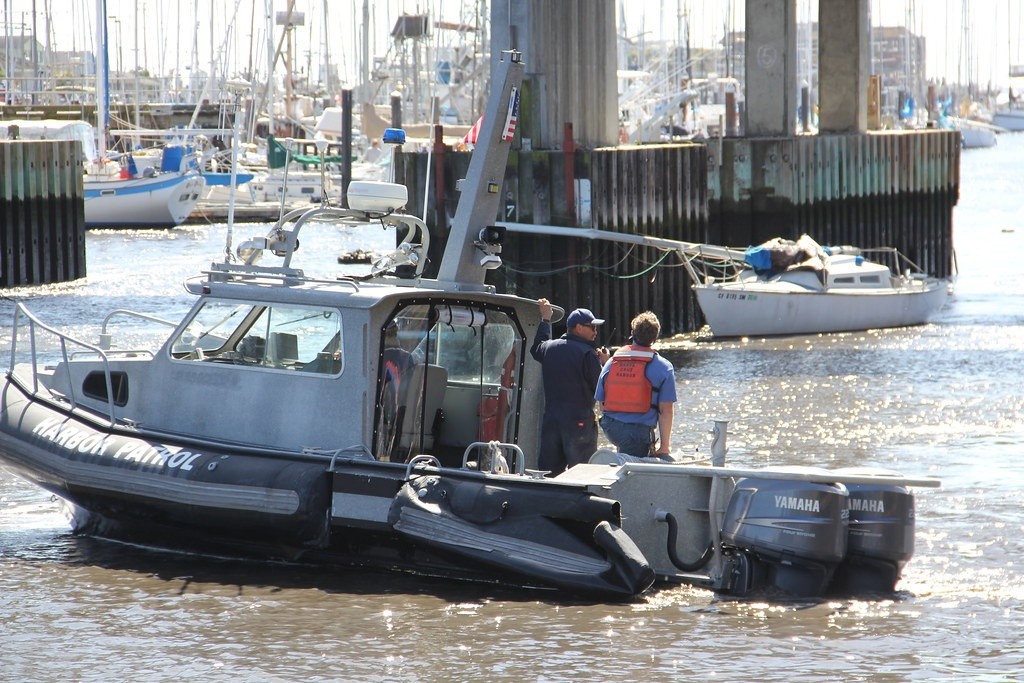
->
[594,311,677,458]
[385,320,402,349]
[529,296,609,477]
[362,139,381,162]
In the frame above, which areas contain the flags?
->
[461,86,521,143]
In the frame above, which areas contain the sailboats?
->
[4,4,1020,233]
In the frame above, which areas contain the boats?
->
[670,232,952,337]
[5,47,943,603]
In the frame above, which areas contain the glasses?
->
[581,324,598,332]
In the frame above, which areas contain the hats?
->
[567,308,605,328]
